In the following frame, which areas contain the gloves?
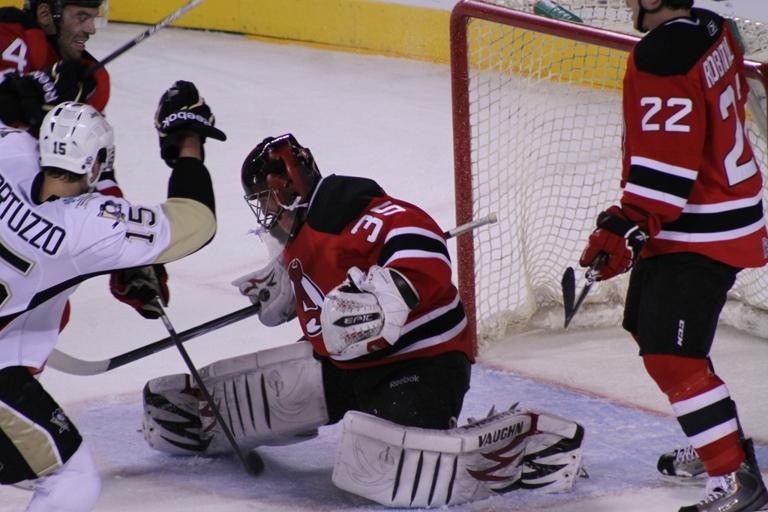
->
[21,58,97,114]
[155,79,226,167]
[110,262,169,319]
[578,206,649,281]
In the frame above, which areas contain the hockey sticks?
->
[45,210,498,376]
[146,287,263,476]
[561,248,607,329]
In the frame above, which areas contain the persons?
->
[140,131,588,510]
[0,0,171,321]
[0,79,228,491]
[577,0,766,511]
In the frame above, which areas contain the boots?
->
[657,444,706,478]
[679,436,768,511]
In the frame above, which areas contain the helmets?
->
[39,101,115,174]
[241,134,319,229]
[23,0,108,29]
